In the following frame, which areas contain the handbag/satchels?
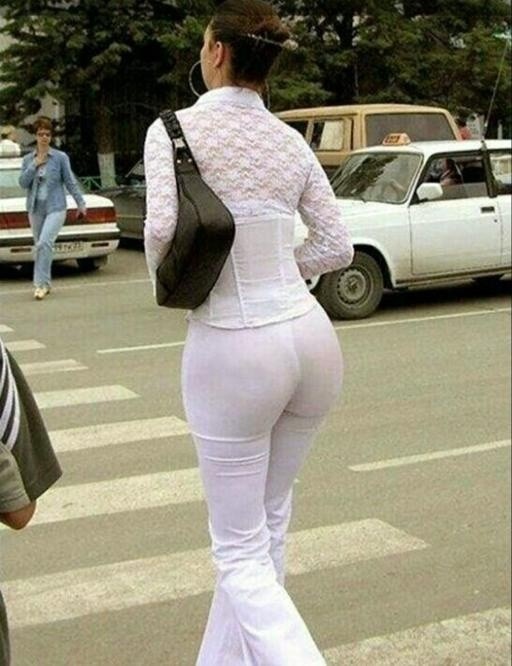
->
[155,148,236,309]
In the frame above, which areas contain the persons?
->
[0,123,22,157]
[144,1,355,666]
[390,158,468,199]
[0,331,61,666]
[19,116,86,299]
[454,117,472,140]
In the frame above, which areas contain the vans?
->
[271,104,467,177]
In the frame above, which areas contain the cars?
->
[93,151,145,247]
[0,136,121,276]
[294,132,512,324]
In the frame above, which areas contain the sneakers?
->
[34,286,51,300]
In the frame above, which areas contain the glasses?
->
[36,133,51,137]
